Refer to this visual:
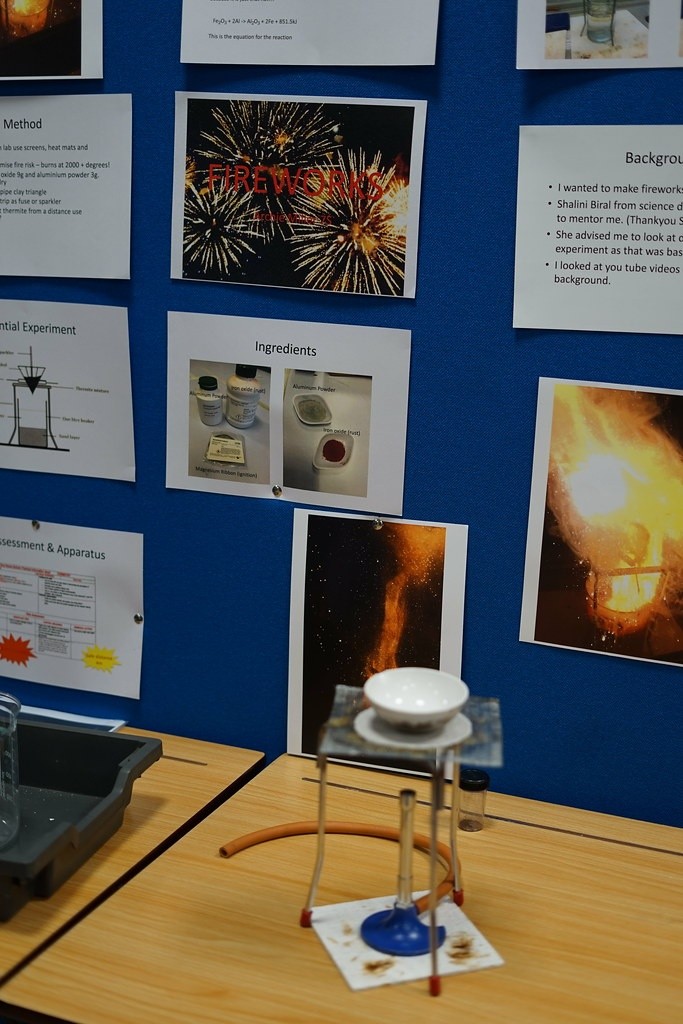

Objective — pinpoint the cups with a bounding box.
[586,0,616,42]
[0,693,24,846]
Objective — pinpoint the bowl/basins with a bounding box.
[365,665,471,735]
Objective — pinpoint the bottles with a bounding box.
[453,767,489,831]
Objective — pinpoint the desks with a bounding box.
[0,698,683,1020]
